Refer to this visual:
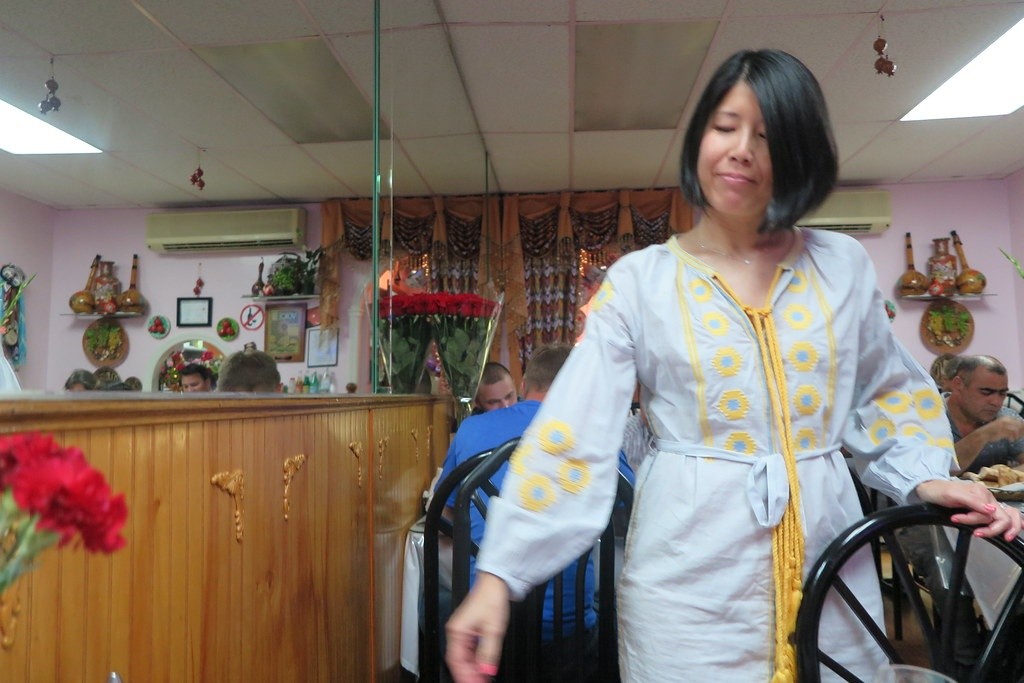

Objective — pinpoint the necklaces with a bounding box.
[695,231,773,264]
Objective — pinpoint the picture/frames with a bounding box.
[175,295,214,329]
[304,324,339,369]
[263,301,308,364]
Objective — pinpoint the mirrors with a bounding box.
[0,0,503,405]
[149,339,229,391]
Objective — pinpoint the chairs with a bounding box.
[790,493,1024,683]
[418,444,617,683]
[453,436,638,683]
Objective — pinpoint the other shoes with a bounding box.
[952,601,986,669]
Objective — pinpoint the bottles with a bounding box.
[91,261,120,314]
[288,367,336,393]
[263,275,276,296]
[927,238,957,296]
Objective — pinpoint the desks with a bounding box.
[403,511,627,683]
[943,481,1024,631]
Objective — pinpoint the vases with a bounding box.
[116,252,148,312]
[927,234,958,294]
[897,230,930,296]
[95,260,119,313]
[67,255,101,313]
[951,224,988,295]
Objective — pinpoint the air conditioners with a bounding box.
[796,189,892,236]
[141,204,307,257]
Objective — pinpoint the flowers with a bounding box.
[366,293,435,394]
[424,290,503,429]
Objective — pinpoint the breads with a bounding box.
[961,463,1024,489]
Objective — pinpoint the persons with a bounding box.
[217,350,282,393]
[64,369,99,392]
[434,342,654,683]
[181,363,213,393]
[98,383,133,391]
[444,49,1024,683]
[894,353,1024,683]
